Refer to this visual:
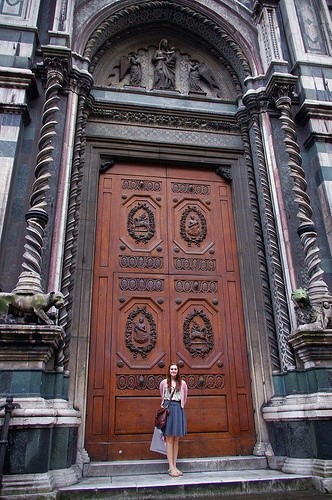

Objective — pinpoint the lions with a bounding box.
[0,291,65,325]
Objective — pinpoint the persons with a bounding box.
[128,38,205,92]
[160,362,188,477]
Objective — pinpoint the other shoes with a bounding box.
[168,467,179,476]
[173,466,183,476]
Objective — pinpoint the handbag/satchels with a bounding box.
[151,426,167,455]
[155,408,170,429]
